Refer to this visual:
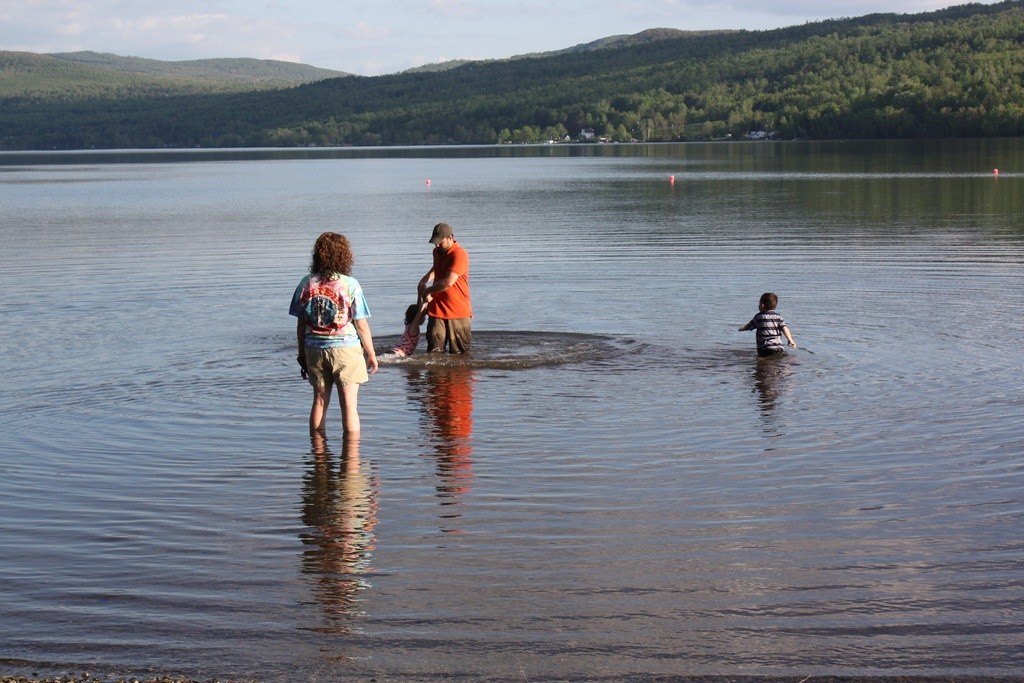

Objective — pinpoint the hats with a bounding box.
[428,222,453,244]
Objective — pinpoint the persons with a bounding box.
[375,283,432,361]
[287,229,381,428]
[736,292,798,358]
[417,223,473,353]
